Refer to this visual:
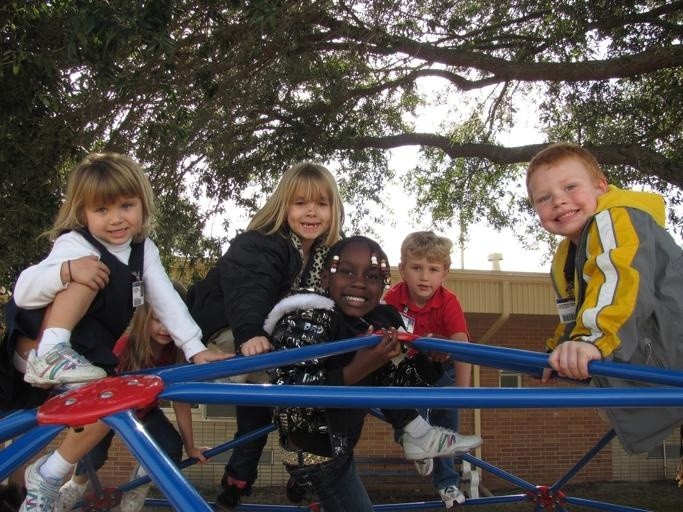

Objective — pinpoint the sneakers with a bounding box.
[120,465,153,512]
[398,426,483,461]
[19,453,61,512]
[56,480,88,512]
[413,459,433,476]
[23,343,106,384]
[215,487,241,512]
[438,485,465,510]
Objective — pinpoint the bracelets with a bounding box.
[65,259,76,284]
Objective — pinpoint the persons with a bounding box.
[380,231,471,510]
[526,144,683,457]
[13,152,236,512]
[188,162,346,512]
[54,279,215,512]
[268,236,482,512]
